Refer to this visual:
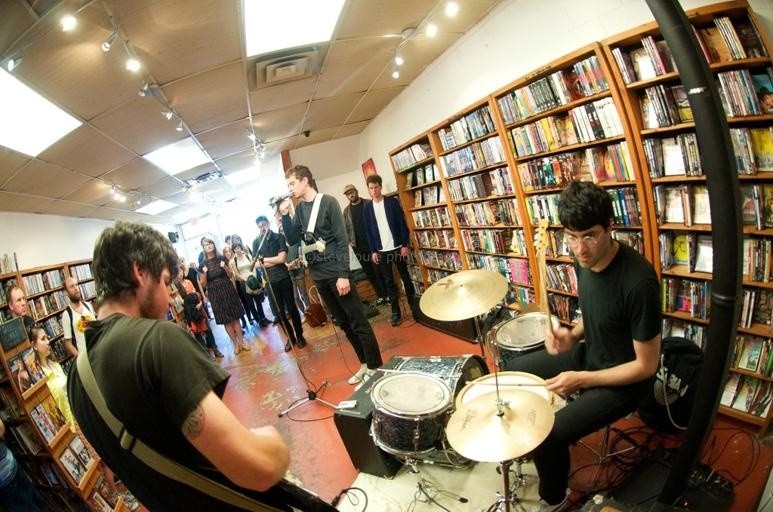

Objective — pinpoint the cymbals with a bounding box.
[419,268,507,321]
[447,389,556,463]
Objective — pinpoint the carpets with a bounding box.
[330,443,546,511]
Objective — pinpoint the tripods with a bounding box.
[260,265,337,417]
[403,466,537,512]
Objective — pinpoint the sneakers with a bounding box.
[375,296,392,307]
[391,313,401,325]
[234,344,251,355]
[207,347,224,361]
[241,317,273,328]
[349,364,378,392]
[530,488,568,512]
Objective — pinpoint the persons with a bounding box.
[27,324,139,511]
[66,221,292,512]
[505,182,664,512]
[172,164,415,392]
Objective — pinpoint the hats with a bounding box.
[343,184,356,194]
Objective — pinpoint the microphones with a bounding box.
[270,192,293,206]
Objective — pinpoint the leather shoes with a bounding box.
[298,337,306,348]
[285,338,296,352]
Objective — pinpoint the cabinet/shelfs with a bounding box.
[389,0,772,437]
[0,254,188,511]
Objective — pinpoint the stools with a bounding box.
[564,386,635,491]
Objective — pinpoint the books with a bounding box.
[0,263,118,512]
[392,0,772,419]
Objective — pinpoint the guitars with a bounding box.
[295,238,326,269]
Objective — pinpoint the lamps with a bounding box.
[386,0,462,84]
[57,2,220,171]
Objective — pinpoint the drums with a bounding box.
[392,355,489,467]
[368,373,451,455]
[494,313,563,359]
[457,371,554,414]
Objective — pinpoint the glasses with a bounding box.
[566,231,603,248]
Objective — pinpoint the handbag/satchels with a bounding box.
[360,299,379,317]
[305,303,327,326]
[637,337,702,429]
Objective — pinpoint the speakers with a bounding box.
[333,354,405,479]
